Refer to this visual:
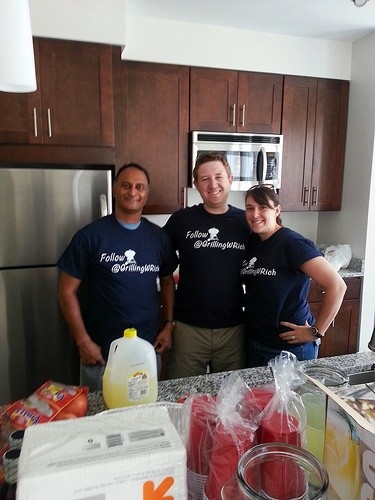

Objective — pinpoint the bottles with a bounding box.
[291,364,349,488]
[103,328,158,409]
[220,442,329,500]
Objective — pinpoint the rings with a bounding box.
[292,336,295,340]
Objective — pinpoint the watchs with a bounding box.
[310,325,324,339]
[164,319,175,328]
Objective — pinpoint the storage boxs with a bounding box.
[14,405,189,500]
[298,370,375,500]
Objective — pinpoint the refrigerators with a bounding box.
[0,168,114,407]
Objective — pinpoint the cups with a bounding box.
[186,387,303,500]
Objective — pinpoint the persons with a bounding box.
[57,162,175,393]
[240,184,346,367]
[161,154,252,380]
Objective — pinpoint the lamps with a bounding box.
[0,0,38,93]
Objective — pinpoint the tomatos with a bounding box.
[51,394,87,420]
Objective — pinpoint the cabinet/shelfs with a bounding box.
[306,277,362,359]
[113,60,351,216]
[0,36,116,171]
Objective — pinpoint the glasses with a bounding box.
[248,183,276,192]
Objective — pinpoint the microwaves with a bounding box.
[188,130,284,192]
